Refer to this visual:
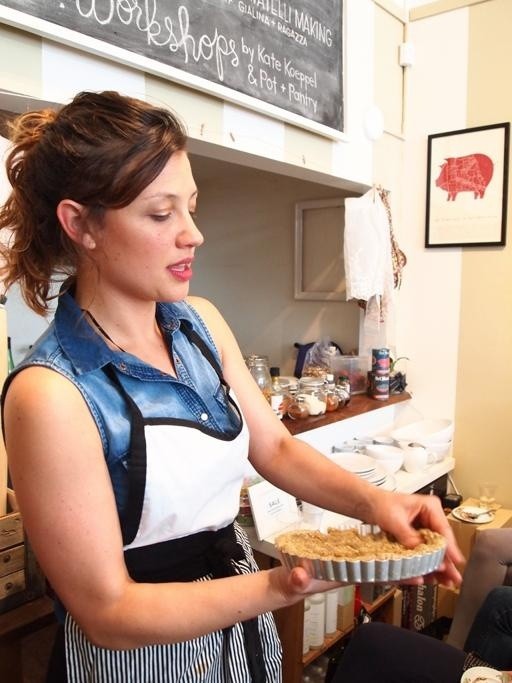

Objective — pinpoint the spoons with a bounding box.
[464,509,499,518]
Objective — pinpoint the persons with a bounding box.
[444,528,512,652]
[324,622,512,683]
[462,587,511,671]
[1,91,467,683]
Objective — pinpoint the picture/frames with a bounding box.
[292,196,354,302]
[423,120,510,248]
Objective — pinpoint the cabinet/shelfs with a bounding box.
[239,452,456,682]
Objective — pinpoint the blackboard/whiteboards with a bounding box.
[1,1,351,140]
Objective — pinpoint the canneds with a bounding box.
[367,348,390,402]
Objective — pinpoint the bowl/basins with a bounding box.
[322,416,453,493]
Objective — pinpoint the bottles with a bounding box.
[1,291,13,397]
[370,347,391,401]
[302,588,337,683]
[248,353,350,420]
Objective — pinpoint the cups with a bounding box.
[332,355,369,395]
[479,495,496,509]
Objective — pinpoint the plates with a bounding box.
[451,505,494,525]
[460,666,502,683]
